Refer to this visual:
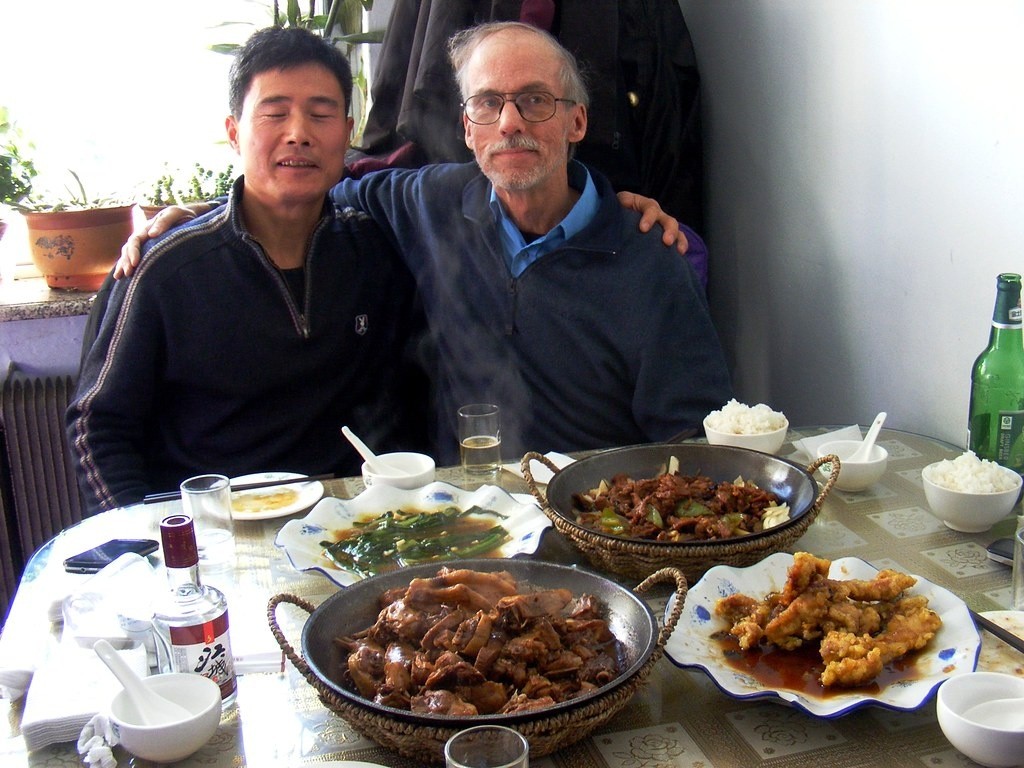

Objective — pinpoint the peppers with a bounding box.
[582,500,748,539]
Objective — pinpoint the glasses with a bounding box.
[460,90,577,125]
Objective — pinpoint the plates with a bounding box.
[273,479,552,590]
[201,471,324,520]
[975,611,1024,682]
[660,552,984,719]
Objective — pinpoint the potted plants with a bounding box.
[142,156,242,222]
[20,179,141,292]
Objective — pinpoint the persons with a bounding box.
[113,19,733,467]
[65,23,689,516]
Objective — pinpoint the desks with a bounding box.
[0,421,1024,768]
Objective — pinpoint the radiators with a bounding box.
[0,376,82,618]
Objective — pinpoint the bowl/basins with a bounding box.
[936,671,1024,768]
[921,462,1023,532]
[361,451,435,489]
[816,440,889,492]
[108,672,222,763]
[703,419,789,456]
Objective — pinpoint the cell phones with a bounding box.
[64,539,160,574]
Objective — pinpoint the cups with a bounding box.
[444,725,529,768]
[457,403,503,474]
[180,475,238,572]
[1010,528,1024,610]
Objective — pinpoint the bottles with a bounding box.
[152,512,238,710]
[965,272,1024,502]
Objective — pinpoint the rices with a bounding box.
[926,449,1019,493]
[706,397,786,434]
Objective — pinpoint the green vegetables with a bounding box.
[319,505,512,577]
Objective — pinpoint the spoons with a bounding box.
[844,412,887,461]
[93,640,192,726]
[341,427,408,477]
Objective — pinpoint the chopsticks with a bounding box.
[968,607,1024,654]
[142,474,334,504]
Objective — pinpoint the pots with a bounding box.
[267,565,688,758]
[518,444,841,585]
